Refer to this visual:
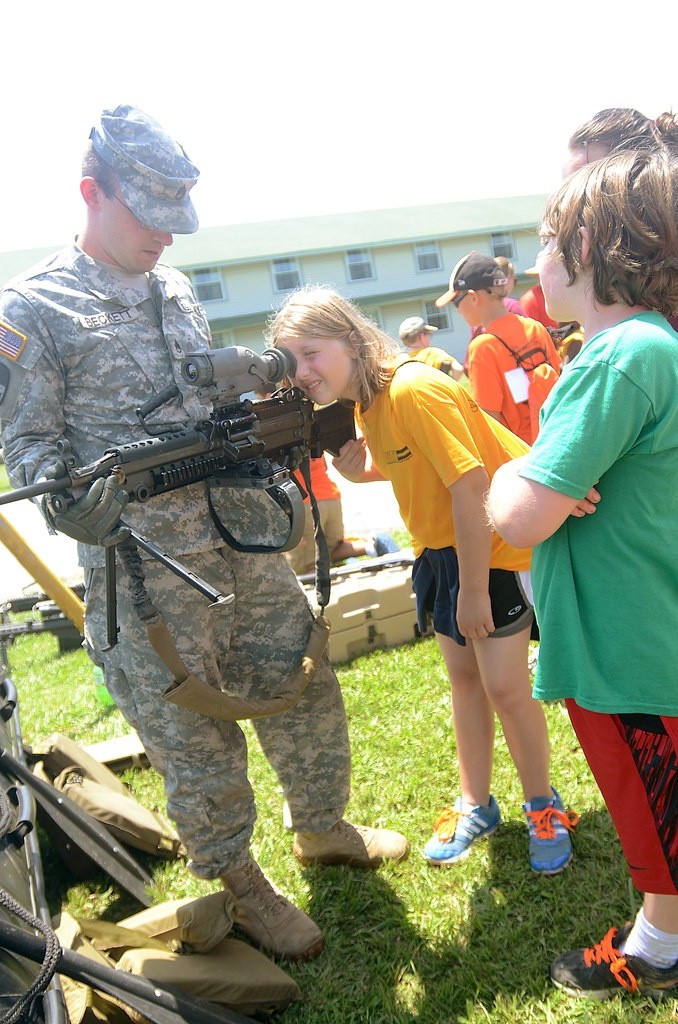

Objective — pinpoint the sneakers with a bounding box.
[425,794,500,864]
[522,787,573,875]
[550,923,678,996]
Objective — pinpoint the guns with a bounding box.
[0,583,84,665]
[0,345,357,652]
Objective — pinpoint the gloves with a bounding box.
[44,466,133,548]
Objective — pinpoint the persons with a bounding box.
[254,378,401,575]
[399,250,583,640]
[483,107,678,1001]
[271,285,579,875]
[0,103,409,964]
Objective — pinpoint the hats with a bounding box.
[434,250,507,309]
[398,317,439,337]
[89,103,200,234]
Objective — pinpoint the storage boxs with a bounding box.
[306,565,436,663]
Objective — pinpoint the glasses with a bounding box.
[94,179,152,232]
[423,332,433,336]
[452,289,479,308]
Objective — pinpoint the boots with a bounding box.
[293,820,412,867]
[221,855,325,959]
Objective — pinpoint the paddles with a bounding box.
[0,745,158,906]
[0,916,254,1024]
